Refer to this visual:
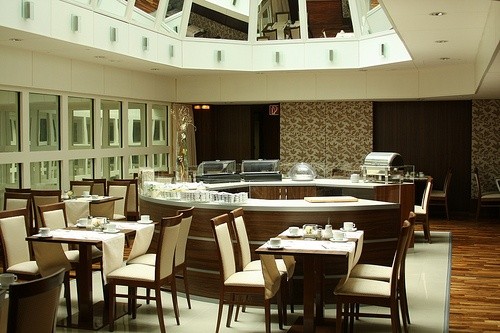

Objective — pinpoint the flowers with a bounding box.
[67,190,74,196]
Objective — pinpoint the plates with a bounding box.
[80,195,91,197]
[329,239,348,242]
[76,223,87,227]
[37,234,52,238]
[137,220,154,224]
[340,228,357,232]
[287,234,301,237]
[267,246,285,249]
[102,230,120,233]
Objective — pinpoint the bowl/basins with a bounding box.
[92,195,98,199]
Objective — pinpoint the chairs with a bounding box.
[474,167,500,216]
[0,178,452,333]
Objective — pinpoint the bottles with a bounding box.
[38,228,50,236]
[288,227,300,236]
[324,225,332,236]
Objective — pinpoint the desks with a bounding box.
[396,177,434,180]
[254,228,365,333]
[25,222,159,331]
[61,195,124,226]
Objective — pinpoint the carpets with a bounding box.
[57,230,451,333]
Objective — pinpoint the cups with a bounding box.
[332,232,345,240]
[140,215,150,222]
[82,191,89,196]
[269,238,282,248]
[343,222,355,231]
[106,224,117,232]
[76,218,88,226]
